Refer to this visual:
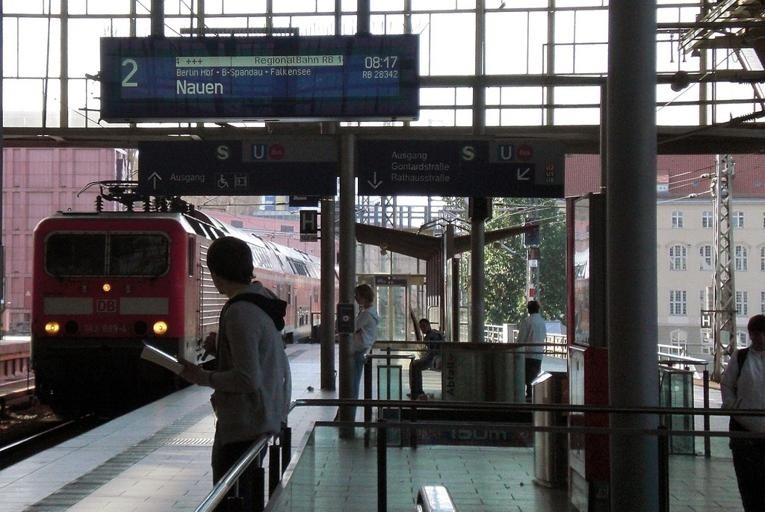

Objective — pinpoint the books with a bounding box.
[137,340,198,385]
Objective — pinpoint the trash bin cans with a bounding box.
[531,371,569,488]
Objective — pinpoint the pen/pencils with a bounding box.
[201,351,209,361]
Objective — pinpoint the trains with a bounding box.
[32,180,339,417]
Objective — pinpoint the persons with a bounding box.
[333,283,379,422]
[513,300,547,403]
[174,234,293,512]
[718,313,765,512]
[405,317,446,401]
